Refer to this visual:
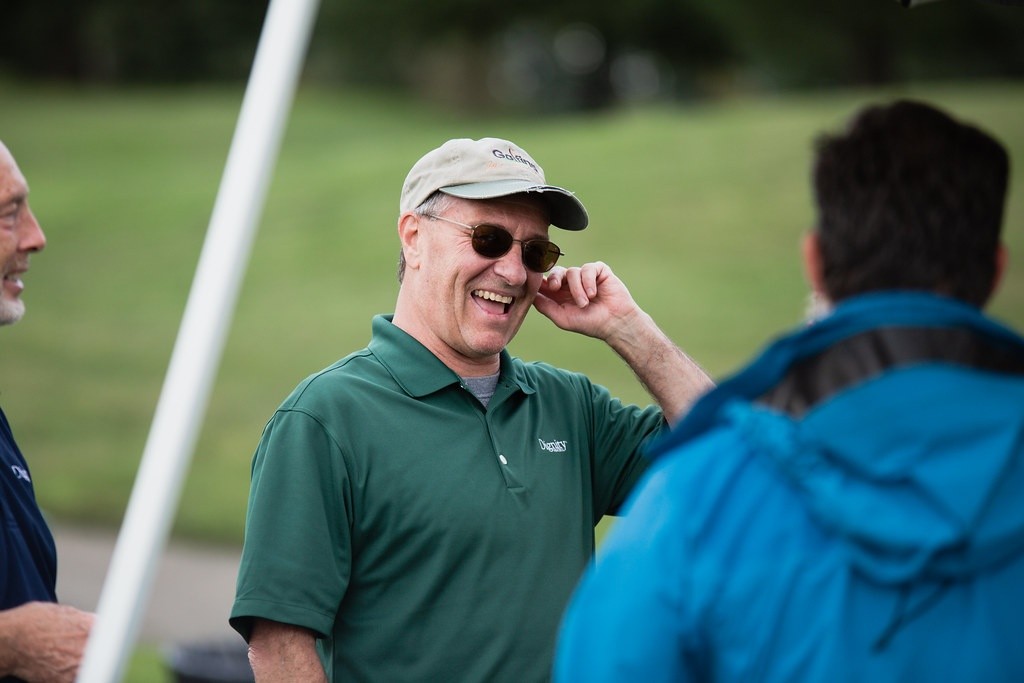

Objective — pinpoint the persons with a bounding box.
[227,138,718,682]
[548,98,1024,682]
[0,141,94,683]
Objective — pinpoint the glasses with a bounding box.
[418,212,566,273]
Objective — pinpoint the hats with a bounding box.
[399,137,588,232]
